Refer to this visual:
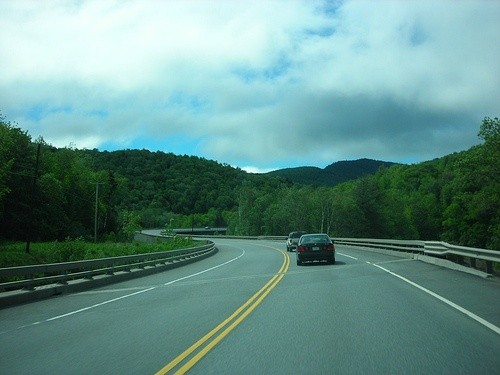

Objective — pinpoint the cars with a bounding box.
[285,231,308,252]
[293,233,335,265]
